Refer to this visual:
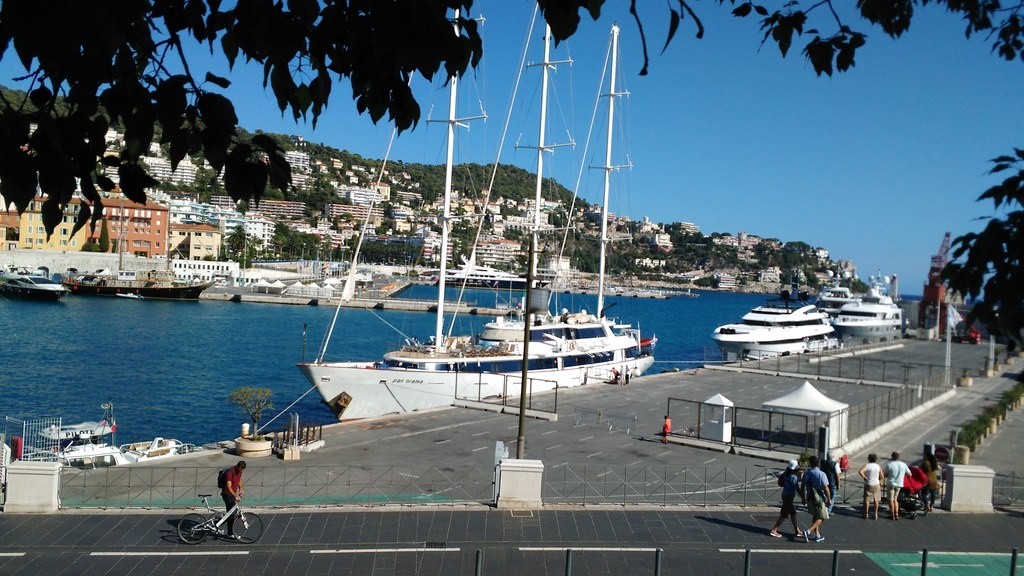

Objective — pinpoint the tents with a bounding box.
[762,381,849,449]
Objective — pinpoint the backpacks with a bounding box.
[218,465,234,488]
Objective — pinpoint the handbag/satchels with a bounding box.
[777,474,785,487]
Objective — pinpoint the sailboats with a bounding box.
[61,199,221,300]
[295,6,655,421]
[474,20,638,346]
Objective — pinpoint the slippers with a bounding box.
[796,532,805,536]
[770,532,782,538]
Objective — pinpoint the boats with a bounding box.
[710,268,841,364]
[0,261,72,302]
[817,272,910,347]
[56,442,131,470]
[38,396,116,439]
[118,437,201,464]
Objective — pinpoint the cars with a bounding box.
[939,324,983,344]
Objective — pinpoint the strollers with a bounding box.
[896,466,930,520]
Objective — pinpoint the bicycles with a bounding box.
[177,494,264,545]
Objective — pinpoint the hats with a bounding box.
[787,459,800,470]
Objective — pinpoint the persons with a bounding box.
[218,461,246,538]
[820,450,840,515]
[411,337,417,345]
[884,451,912,522]
[770,459,806,537]
[919,453,942,512]
[662,415,672,443]
[801,456,833,543]
[612,365,641,384]
[859,453,884,520]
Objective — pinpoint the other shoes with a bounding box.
[897,515,901,520]
[816,536,825,542]
[803,529,810,542]
[228,535,241,539]
[888,515,896,520]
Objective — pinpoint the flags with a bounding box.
[947,304,964,331]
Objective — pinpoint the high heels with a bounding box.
[924,505,933,511]
